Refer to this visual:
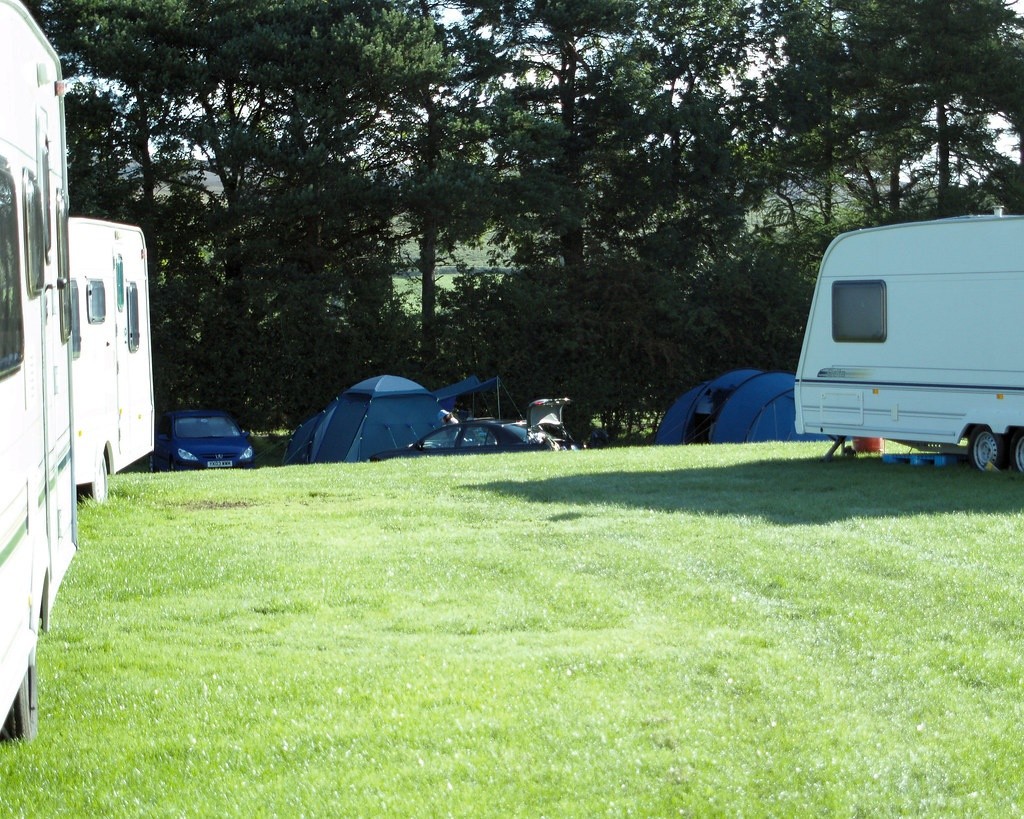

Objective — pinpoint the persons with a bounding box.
[437,410,459,426]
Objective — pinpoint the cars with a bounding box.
[364,418,588,466]
[148,409,255,473]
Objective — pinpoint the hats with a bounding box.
[438,409,449,420]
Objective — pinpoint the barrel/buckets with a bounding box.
[852,436,881,453]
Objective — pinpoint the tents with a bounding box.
[282,375,453,464]
[652,368,853,446]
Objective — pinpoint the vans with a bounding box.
[0,0,83,743]
[793,204,1024,475]
[62,217,156,510]
